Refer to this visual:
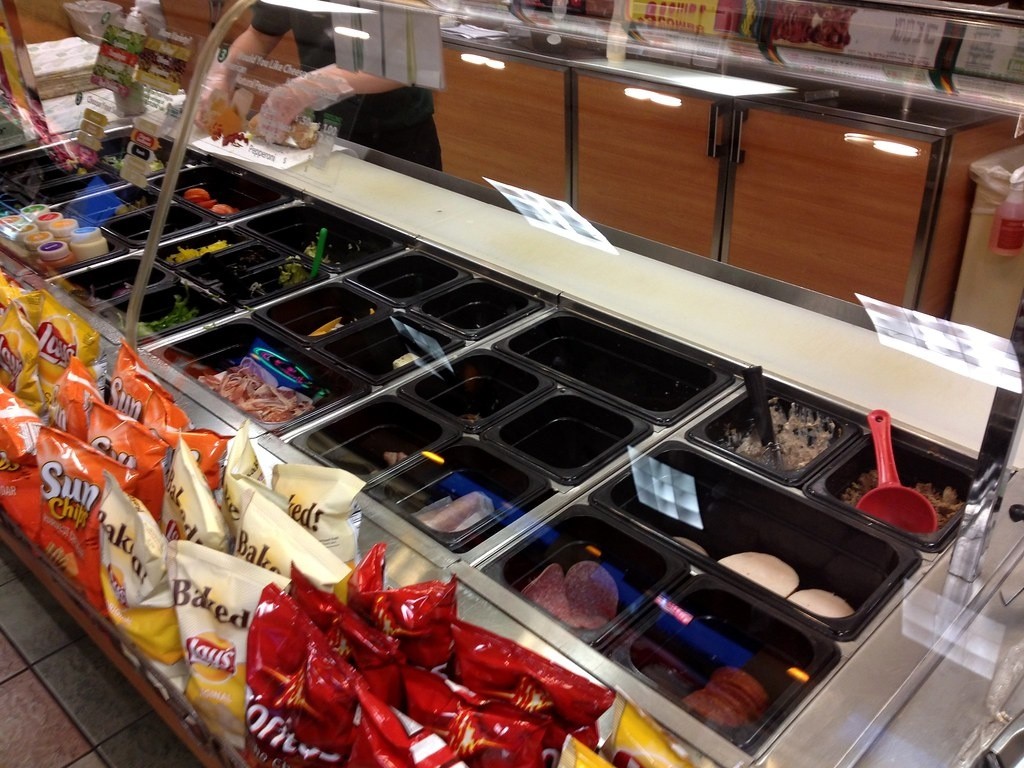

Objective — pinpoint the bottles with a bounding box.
[0,204,112,272]
[989,164,1024,261]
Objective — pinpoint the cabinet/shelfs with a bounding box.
[160,1,325,131]
[428,20,606,216]
[574,45,1024,316]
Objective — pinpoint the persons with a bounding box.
[189,0,444,178]
